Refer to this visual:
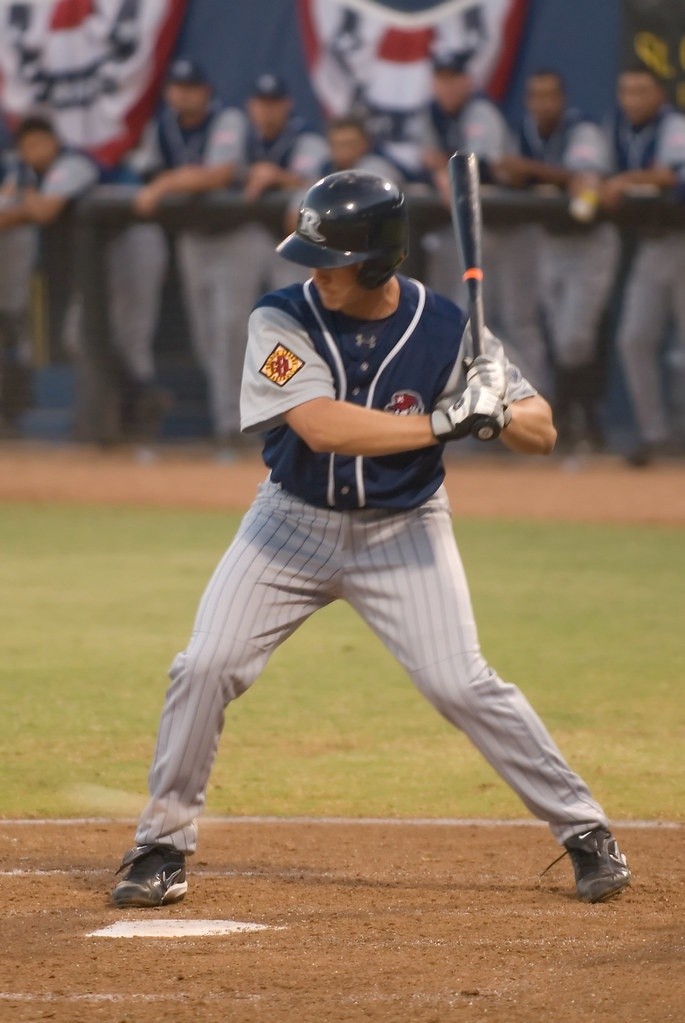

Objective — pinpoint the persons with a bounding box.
[110,170,631,908]
[0,36,685,462]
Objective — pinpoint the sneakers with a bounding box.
[113,843,188,906]
[539,825,632,903]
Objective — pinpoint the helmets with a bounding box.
[275,168,411,289]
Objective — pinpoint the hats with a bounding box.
[254,69,287,100]
[162,60,206,83]
[434,52,465,73]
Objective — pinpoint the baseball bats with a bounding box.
[447,149,501,442]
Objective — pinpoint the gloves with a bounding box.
[429,383,504,443]
[462,354,511,428]
